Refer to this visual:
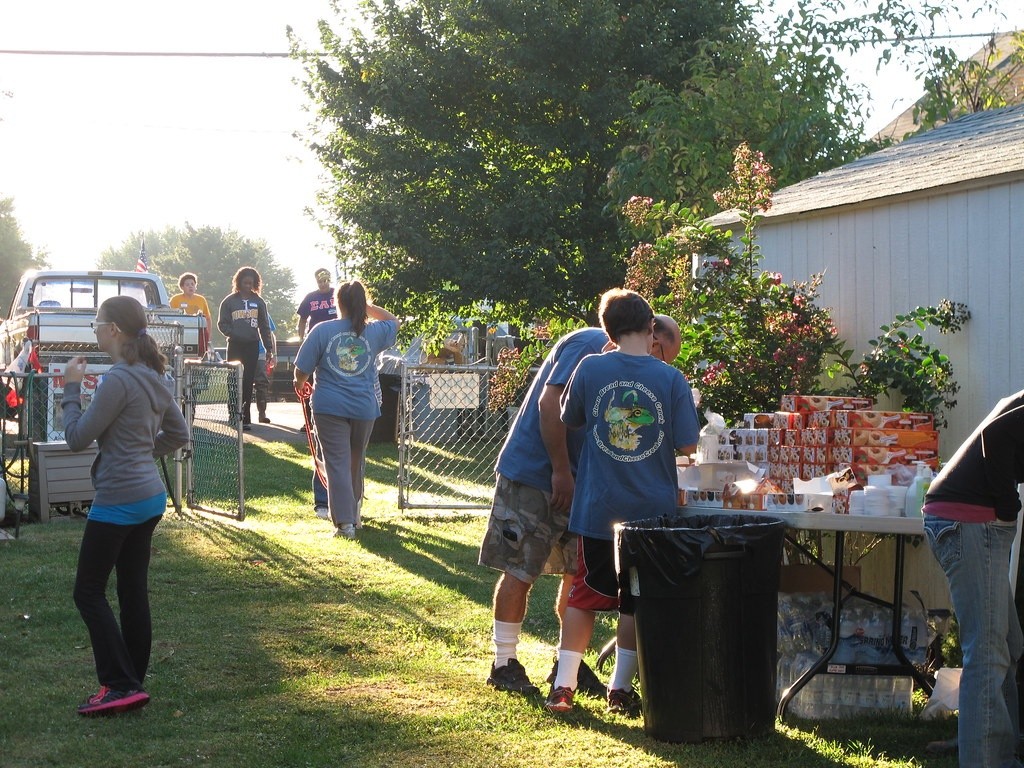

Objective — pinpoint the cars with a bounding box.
[191,339,309,403]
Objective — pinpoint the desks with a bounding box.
[683,507,933,722]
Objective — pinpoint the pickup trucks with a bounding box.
[1,269,210,426]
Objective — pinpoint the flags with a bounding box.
[135,239,149,273]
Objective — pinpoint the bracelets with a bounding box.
[273,353,278,356]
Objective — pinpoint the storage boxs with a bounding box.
[681,394,938,511]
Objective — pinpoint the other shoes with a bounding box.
[300,424,313,432]
[333,525,357,540]
[259,417,270,423]
[243,424,252,430]
[316,506,329,519]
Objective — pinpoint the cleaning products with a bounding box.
[904,459,936,518]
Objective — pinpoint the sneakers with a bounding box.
[545,683,578,713]
[75,686,151,718]
[486,658,540,696]
[607,686,643,715]
[545,656,612,698]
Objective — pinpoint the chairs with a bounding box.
[39,300,61,306]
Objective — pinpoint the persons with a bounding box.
[170,269,338,432]
[479,313,681,706]
[544,287,700,712]
[292,279,400,541]
[920,387,1024,768]
[63,297,190,715]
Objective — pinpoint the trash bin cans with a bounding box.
[613,510,786,744]
[371,373,402,442]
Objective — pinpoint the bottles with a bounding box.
[775,586,930,718]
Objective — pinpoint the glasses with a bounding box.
[90,321,121,334]
